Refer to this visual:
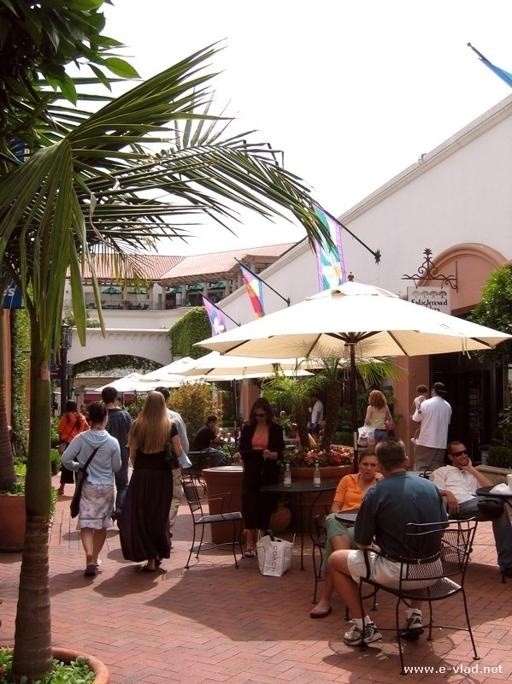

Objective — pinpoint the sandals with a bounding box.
[244,548,255,557]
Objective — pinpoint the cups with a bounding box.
[506,473,512,489]
[227,432,231,442]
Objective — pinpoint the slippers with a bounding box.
[83,561,101,577]
[309,600,333,619]
[144,559,163,572]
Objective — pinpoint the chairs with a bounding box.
[179,440,511,677]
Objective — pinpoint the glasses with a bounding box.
[451,450,468,457]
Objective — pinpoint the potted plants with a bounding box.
[0,1,337,684]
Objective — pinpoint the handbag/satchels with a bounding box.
[257,529,297,578]
[384,416,396,431]
[70,483,81,517]
[476,492,506,514]
[163,441,180,468]
[58,442,67,452]
[357,426,376,445]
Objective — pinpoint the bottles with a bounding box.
[283,463,292,487]
[312,461,321,487]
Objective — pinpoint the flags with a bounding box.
[241,268,265,317]
[202,298,226,338]
[301,204,346,292]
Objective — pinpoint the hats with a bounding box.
[433,382,447,393]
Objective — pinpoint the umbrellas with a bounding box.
[143,355,219,416]
[103,371,168,397]
[166,344,395,424]
[188,279,511,428]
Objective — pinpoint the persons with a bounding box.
[409,384,430,438]
[192,414,225,466]
[118,390,183,571]
[79,401,88,416]
[309,389,325,431]
[411,381,452,470]
[97,385,131,526]
[310,447,384,616]
[151,386,193,539]
[59,399,88,499]
[279,410,288,418]
[364,390,393,440]
[232,396,285,556]
[431,439,511,578]
[64,407,123,576]
[327,436,449,645]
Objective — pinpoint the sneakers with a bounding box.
[342,621,384,647]
[400,609,426,637]
